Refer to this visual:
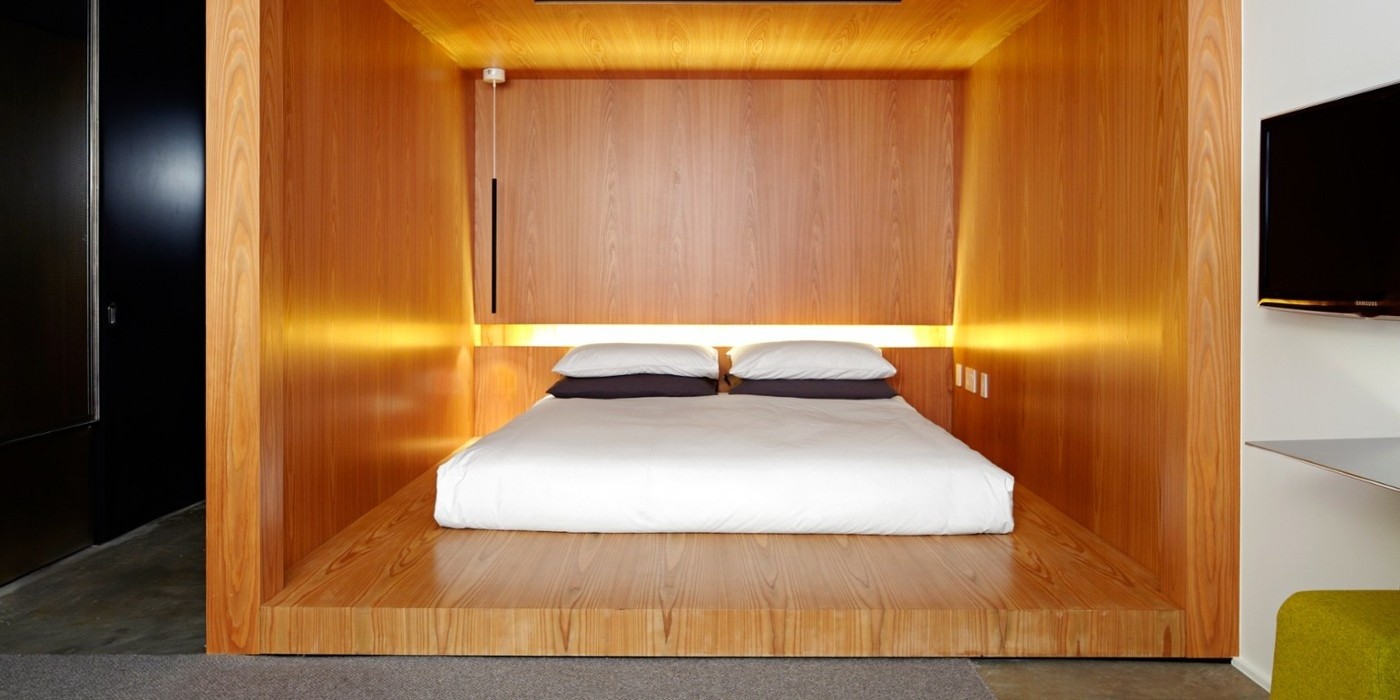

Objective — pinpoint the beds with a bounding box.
[433,344,1015,535]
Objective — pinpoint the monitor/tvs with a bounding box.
[1260,79,1400,319]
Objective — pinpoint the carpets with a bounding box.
[0,653,998,700]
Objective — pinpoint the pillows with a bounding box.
[545,376,718,398]
[725,339,897,380]
[728,378,899,399]
[552,344,720,379]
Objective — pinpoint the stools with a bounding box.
[1269,590,1400,700]
